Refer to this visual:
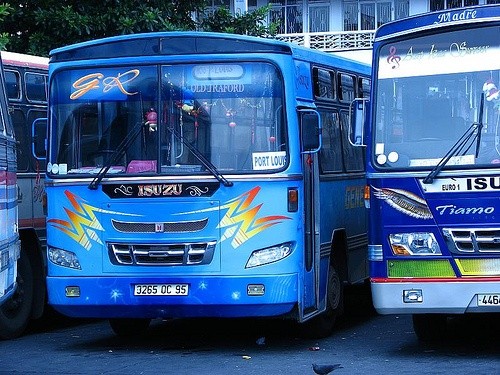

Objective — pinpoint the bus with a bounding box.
[31,30,371,339]
[348,2,500,339]
[0,50,51,339]
